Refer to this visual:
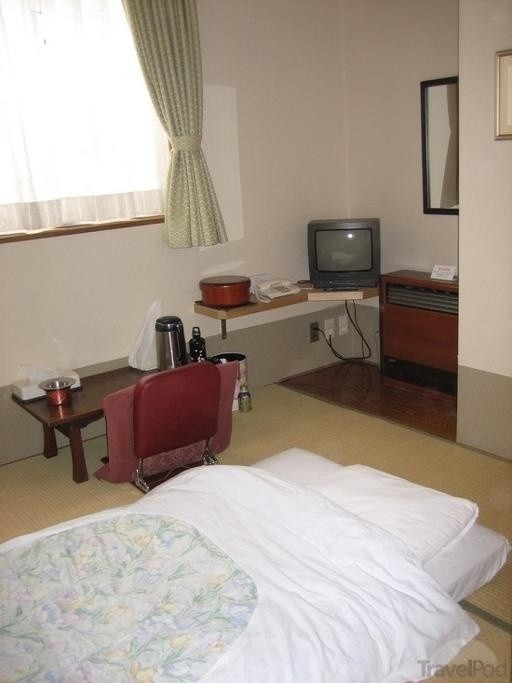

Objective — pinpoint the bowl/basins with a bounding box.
[37,376,78,407]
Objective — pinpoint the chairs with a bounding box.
[134,360,222,493]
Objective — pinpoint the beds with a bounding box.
[0,446,510,682]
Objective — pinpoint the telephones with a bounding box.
[254,277,300,303]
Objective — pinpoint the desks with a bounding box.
[11,352,220,484]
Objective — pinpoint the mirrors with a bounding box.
[421,76,459,214]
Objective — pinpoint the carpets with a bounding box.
[275,358,457,443]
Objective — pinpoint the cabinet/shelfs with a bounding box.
[380,269,458,398]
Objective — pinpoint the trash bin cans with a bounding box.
[210,353,247,412]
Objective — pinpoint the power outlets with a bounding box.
[310,318,336,342]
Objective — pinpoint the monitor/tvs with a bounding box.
[307,217,380,291]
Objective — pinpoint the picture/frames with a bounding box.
[495,49,512,140]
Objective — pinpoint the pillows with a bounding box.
[310,464,480,565]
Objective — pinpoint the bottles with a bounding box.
[237,384,252,412]
[154,314,188,372]
[188,326,207,365]
